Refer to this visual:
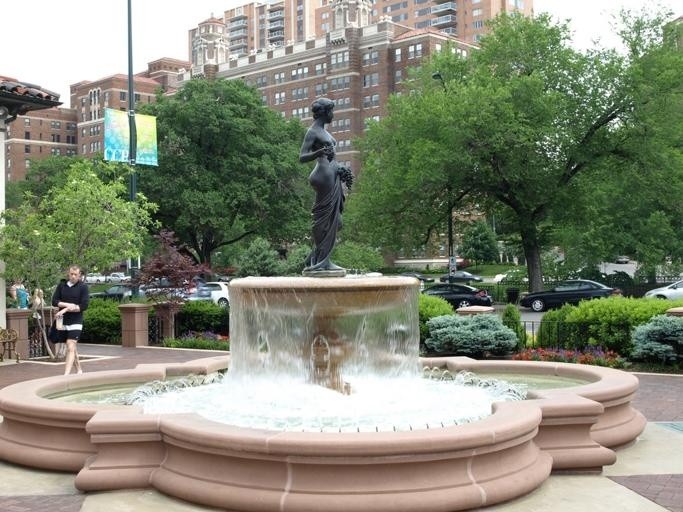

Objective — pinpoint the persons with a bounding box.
[298,95,354,272]
[29,288,46,348]
[11,275,28,309]
[50,264,89,375]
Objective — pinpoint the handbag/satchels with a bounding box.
[49,314,67,344]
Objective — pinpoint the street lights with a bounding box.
[431,72,456,283]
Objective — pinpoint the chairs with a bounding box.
[0,327,21,363]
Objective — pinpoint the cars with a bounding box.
[81,272,229,308]
[397,270,495,310]
[519,256,683,312]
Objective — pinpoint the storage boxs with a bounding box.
[505,287,520,305]
